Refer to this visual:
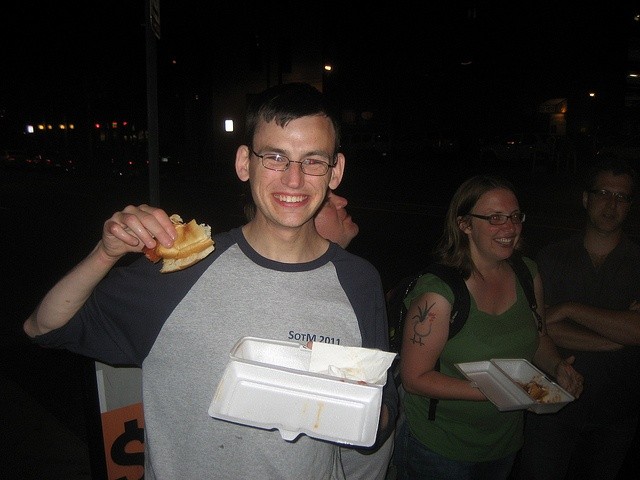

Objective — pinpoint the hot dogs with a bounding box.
[141,213,215,273]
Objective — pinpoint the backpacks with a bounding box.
[386,245,545,422]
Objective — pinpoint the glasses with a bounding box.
[588,189,630,204]
[464,211,525,226]
[250,147,338,177]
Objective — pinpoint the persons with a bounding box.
[392,173,585,480]
[23,83,399,479]
[521,156,639,479]
[229,170,408,479]
